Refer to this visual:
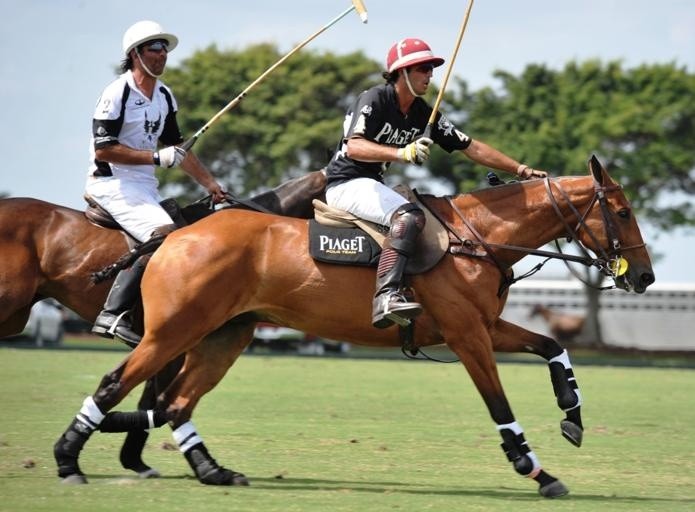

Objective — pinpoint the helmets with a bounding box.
[387,38,444,73]
[122,21,178,56]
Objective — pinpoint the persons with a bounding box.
[321,36,553,330]
[82,17,229,348]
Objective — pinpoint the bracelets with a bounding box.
[517,163,528,177]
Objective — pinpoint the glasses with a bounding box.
[142,41,168,52]
[400,64,433,72]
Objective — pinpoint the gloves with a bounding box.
[398,137,433,163]
[154,145,186,168]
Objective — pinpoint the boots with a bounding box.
[92,224,183,348]
[372,202,424,328]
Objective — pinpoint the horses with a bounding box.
[0,167,327,479]
[53,154,655,498]
